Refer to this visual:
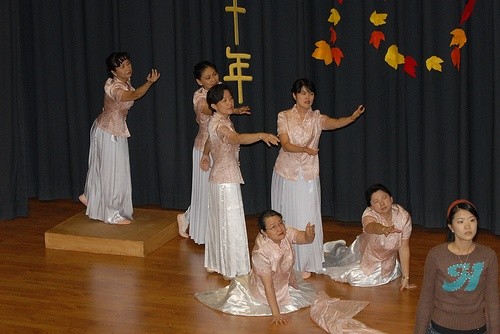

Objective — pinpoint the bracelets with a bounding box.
[402,276,409,280]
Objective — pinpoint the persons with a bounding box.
[192,210,318,326]
[197,85,281,281]
[269,78,365,277]
[413,200,500,334]
[79,53,160,224]
[321,184,417,292]
[177,60,251,245]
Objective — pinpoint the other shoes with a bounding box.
[323,239,346,253]
[79,194,88,207]
[116,218,132,225]
[302,272,312,279]
[177,214,189,238]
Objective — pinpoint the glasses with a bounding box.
[265,220,285,231]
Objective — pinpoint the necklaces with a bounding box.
[455,244,470,270]
